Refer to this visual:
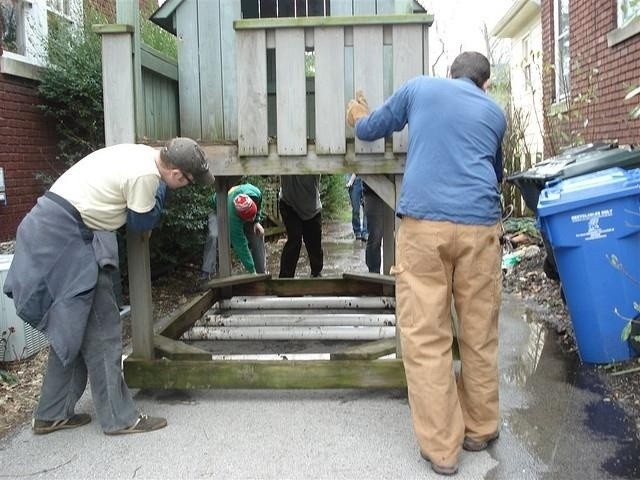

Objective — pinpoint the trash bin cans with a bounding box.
[506,141,640,365]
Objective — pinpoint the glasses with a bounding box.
[182,172,196,185]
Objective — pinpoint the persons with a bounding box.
[346,175,368,244]
[1,136,218,440]
[200,183,270,282]
[275,176,329,281]
[360,173,384,275]
[345,50,508,480]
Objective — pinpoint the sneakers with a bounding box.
[104,414,167,433]
[462,427,500,450]
[421,450,459,474]
[356,232,368,240]
[33,413,90,432]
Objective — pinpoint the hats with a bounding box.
[234,194,257,219]
[166,138,215,185]
